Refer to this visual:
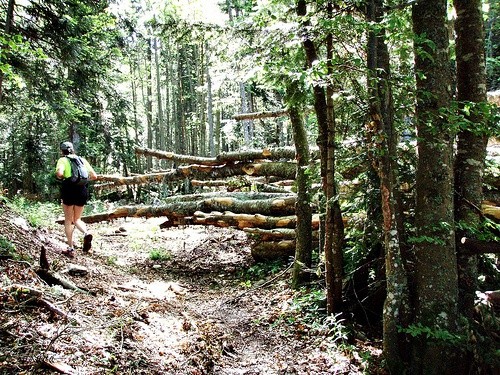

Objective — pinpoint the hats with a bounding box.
[61,142,73,151]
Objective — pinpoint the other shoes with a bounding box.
[82,232,93,252]
[62,247,75,256]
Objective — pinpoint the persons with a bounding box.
[55,141,97,258]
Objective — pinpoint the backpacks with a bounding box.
[63,154,90,186]
